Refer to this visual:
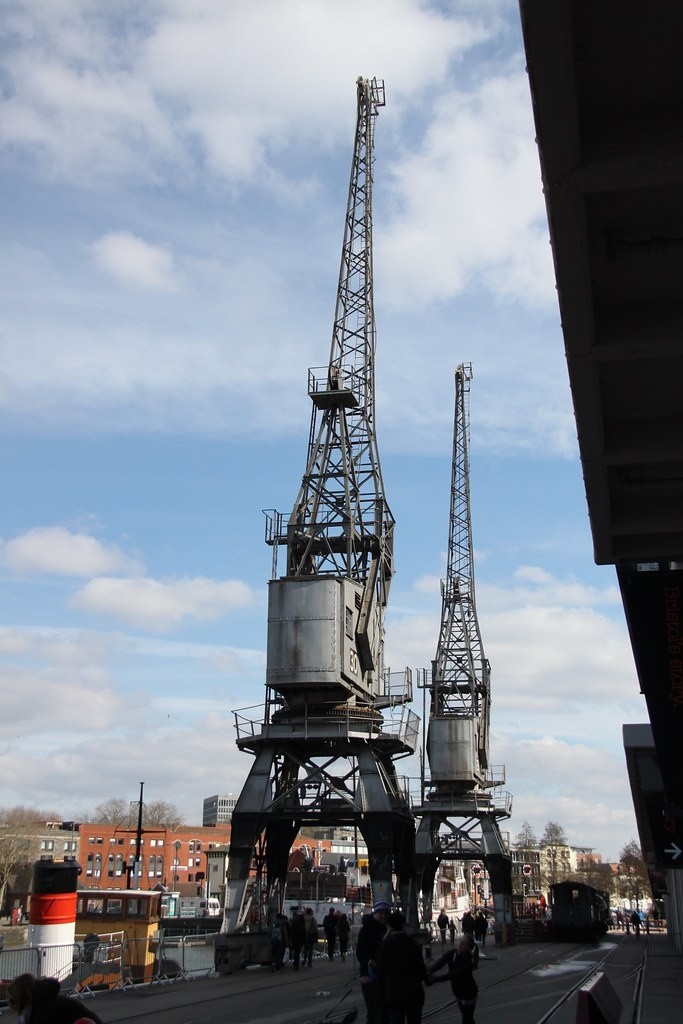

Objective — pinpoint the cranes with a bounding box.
[207,74,418,974]
[403,360,521,949]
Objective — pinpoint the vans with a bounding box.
[195,898,221,917]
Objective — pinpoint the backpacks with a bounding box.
[270,926,282,942]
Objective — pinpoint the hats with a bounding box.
[387,910,407,931]
[374,898,392,913]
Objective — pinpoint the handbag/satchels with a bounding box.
[366,965,382,999]
[308,933,318,943]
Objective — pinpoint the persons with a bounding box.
[356,899,430,1024]
[449,920,458,944]
[539,893,546,924]
[457,912,488,947]
[437,909,450,944]
[323,908,350,961]
[7,974,104,1024]
[271,906,319,970]
[17,904,24,925]
[426,934,479,1024]
[607,910,651,936]
[339,856,346,872]
